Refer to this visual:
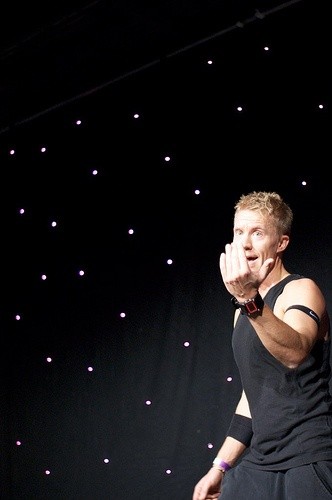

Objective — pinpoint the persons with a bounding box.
[190,190,332,500]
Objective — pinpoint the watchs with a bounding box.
[230,290,264,319]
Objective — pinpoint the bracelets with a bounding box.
[212,458,231,471]
